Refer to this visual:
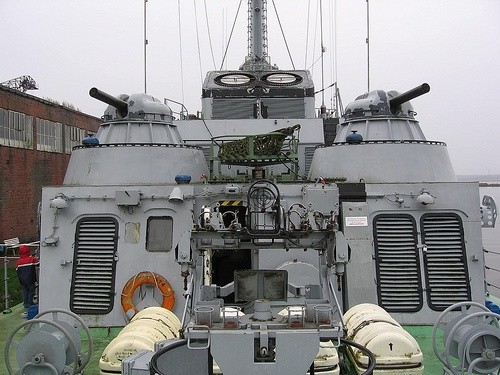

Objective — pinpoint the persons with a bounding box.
[15,245,40,308]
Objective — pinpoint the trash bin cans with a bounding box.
[28,306,38,320]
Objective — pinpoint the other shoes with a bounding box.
[23,302,36,307]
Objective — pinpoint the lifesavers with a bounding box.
[120,270,175,320]
